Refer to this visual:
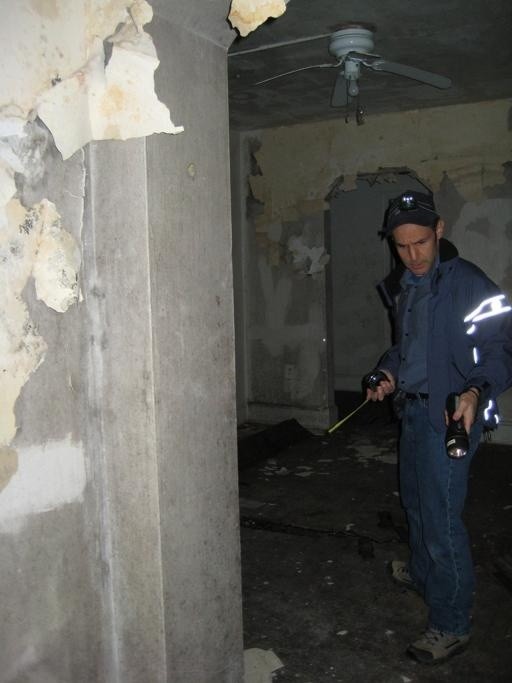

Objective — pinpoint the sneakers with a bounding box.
[406,626,470,665]
[387,559,420,591]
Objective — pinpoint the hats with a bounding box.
[384,189,439,239]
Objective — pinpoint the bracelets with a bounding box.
[470,389,479,399]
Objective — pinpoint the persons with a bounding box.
[359,188,511,668]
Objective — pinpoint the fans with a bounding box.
[252,26,456,108]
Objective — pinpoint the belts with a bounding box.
[400,392,428,401]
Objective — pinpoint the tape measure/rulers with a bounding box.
[327,371,389,434]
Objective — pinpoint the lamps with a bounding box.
[344,57,360,96]
